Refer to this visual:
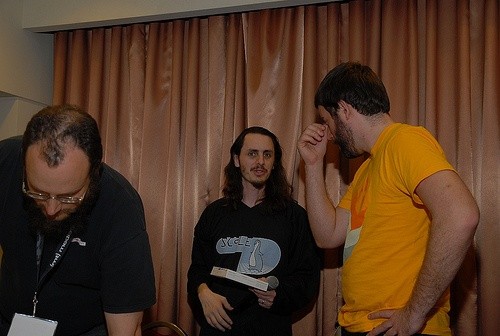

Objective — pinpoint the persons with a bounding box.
[296,61,480,336]
[186,126,323,336]
[0,104,156,336]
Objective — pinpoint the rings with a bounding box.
[261,299,265,305]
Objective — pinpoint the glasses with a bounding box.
[22,166,94,204]
